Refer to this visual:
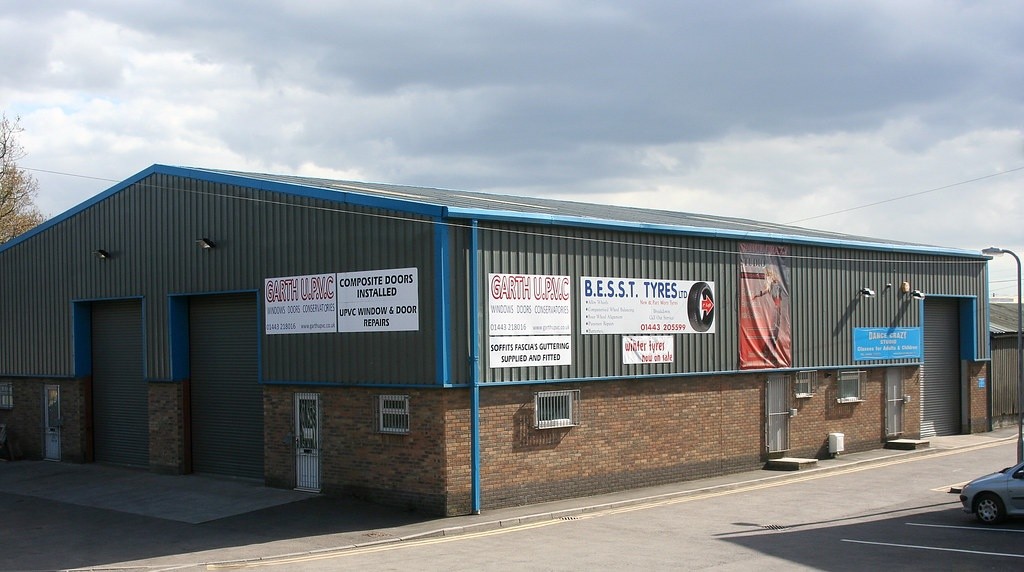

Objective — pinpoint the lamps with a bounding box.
[91,249,110,259]
[860,288,875,298]
[910,290,925,299]
[195,238,216,250]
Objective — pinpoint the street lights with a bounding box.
[983,247,1024,465]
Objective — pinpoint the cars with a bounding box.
[960,461,1024,524]
